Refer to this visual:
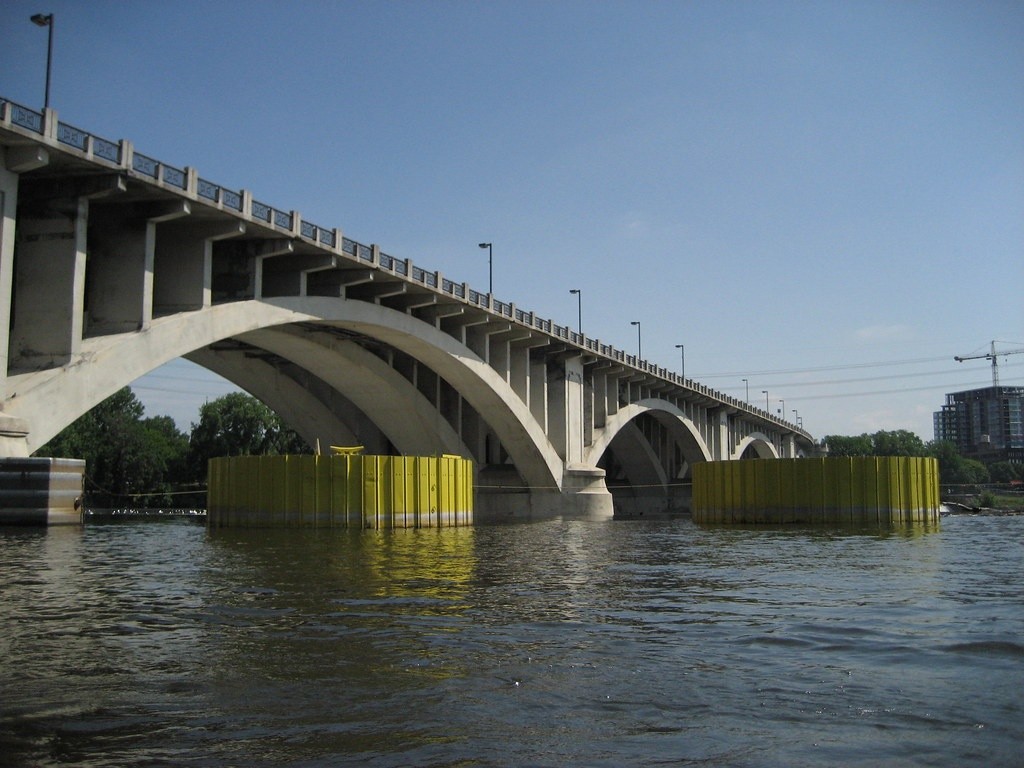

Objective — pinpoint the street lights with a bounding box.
[792,409,802,428]
[29,11,55,108]
[778,400,785,420]
[742,378,749,404]
[569,289,581,334]
[675,344,685,377]
[630,322,642,359]
[478,241,494,293]
[761,390,769,412]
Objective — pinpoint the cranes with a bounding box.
[953,339,1024,386]
